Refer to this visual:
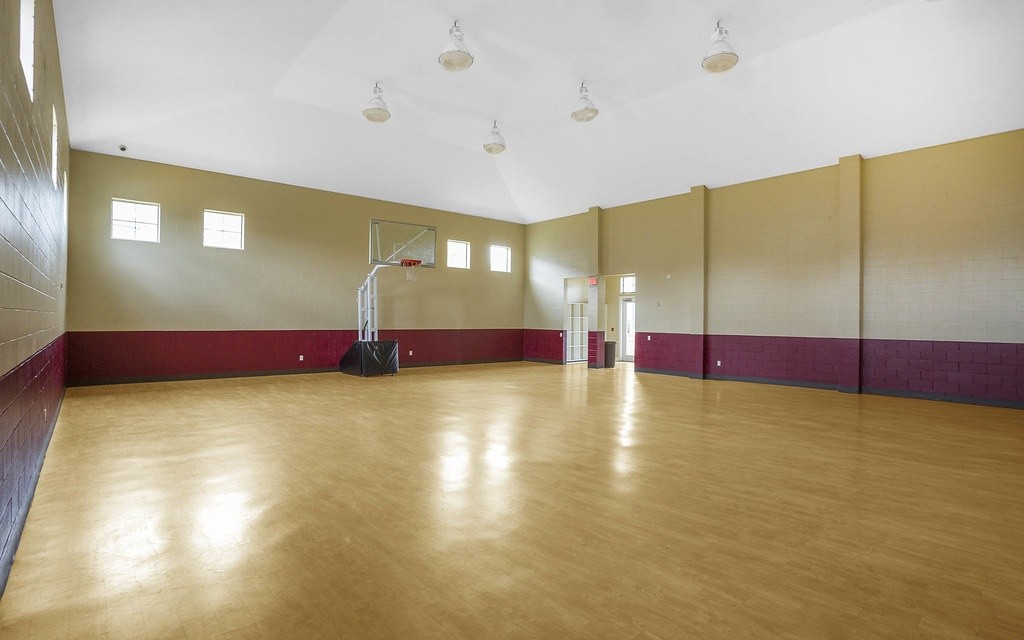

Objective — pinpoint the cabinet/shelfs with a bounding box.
[567,303,589,362]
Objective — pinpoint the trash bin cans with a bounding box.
[604,340,618,368]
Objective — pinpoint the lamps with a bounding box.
[362,82,391,122]
[483,121,506,153]
[438,20,473,71]
[701,21,740,72]
[570,81,599,121]
